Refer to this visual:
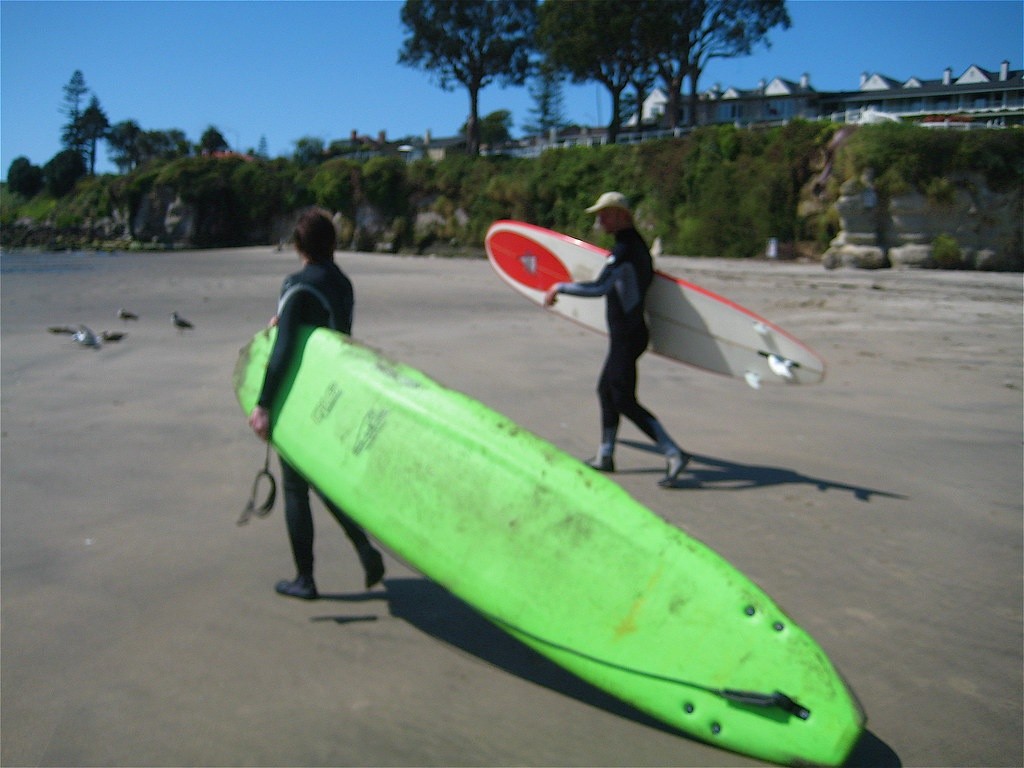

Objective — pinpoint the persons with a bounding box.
[248,208,384,599]
[544,192,690,487]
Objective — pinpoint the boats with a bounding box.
[482,218,828,393]
[232,324,870,768]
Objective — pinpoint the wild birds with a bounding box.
[170,310,195,335]
[117,308,139,327]
[99,330,128,344]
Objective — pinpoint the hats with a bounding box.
[585,191,628,214]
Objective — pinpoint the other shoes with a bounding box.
[584,456,614,471]
[365,549,385,586]
[657,450,693,486]
[277,580,317,600]
